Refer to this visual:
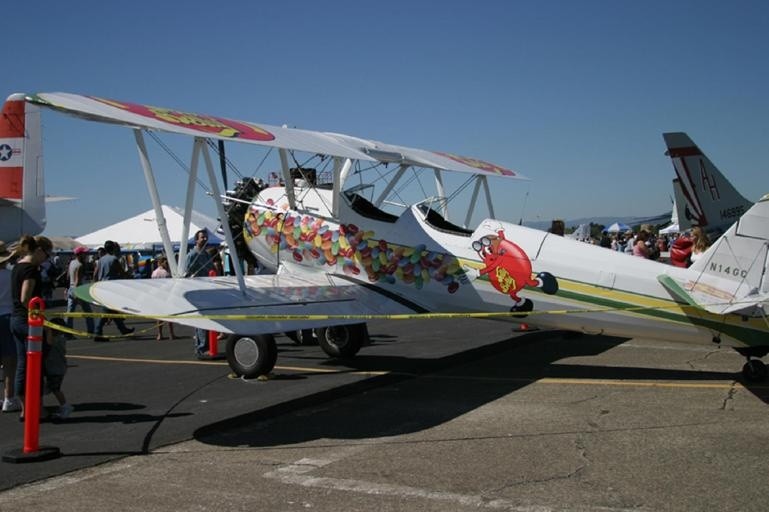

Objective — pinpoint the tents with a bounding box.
[153,226,229,260]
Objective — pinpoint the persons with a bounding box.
[63,240,177,340]
[1,234,73,422]
[184,230,228,362]
[550,219,715,269]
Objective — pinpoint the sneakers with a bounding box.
[19,413,59,423]
[2,399,20,412]
[59,403,74,419]
[94,336,109,342]
[121,327,135,335]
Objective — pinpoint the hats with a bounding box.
[74,245,93,255]
[0,240,18,264]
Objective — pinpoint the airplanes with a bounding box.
[25,90,769,380]
[0,91,47,244]
[661,131,757,249]
